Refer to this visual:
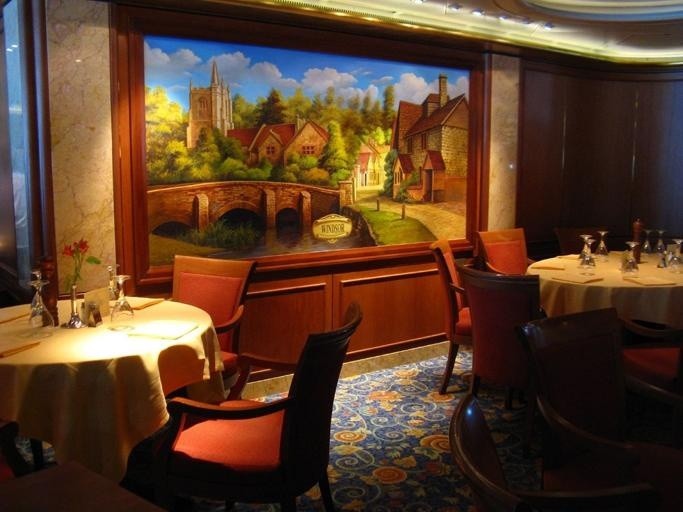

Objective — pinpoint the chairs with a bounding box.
[166,253,258,402]
[430,229,546,393]
[2,418,162,511]
[449,391,663,510]
[171,298,363,511]
[621,344,682,407]
[519,307,682,509]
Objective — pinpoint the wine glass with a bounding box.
[596,230,611,262]
[578,233,596,275]
[659,238,683,274]
[103,264,136,331]
[622,241,640,282]
[641,229,664,253]
[24,268,54,338]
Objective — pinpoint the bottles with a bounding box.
[633,218,641,265]
[78,302,103,327]
[38,257,60,326]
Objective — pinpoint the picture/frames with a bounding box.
[129,3,483,286]
